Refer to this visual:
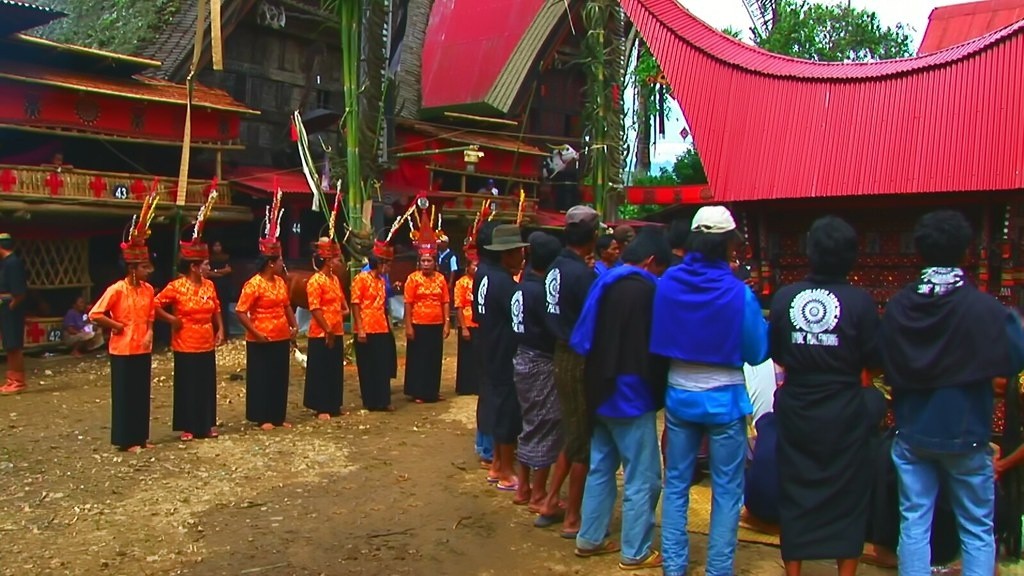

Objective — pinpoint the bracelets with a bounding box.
[293,327,298,331]
[445,318,451,321]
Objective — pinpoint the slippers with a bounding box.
[619,549,663,569]
[487,474,500,481]
[534,508,563,527]
[496,476,532,490]
[513,494,547,504]
[575,540,620,556]
[561,526,610,539]
[480,461,492,468]
[180,431,218,442]
[527,500,564,513]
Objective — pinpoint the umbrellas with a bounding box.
[299,108,343,151]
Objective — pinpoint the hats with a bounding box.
[317,179,341,259]
[259,177,285,256]
[482,223,531,250]
[179,175,219,260]
[435,212,449,242]
[373,190,424,260]
[120,176,161,263]
[528,231,558,269]
[463,199,495,262]
[692,205,746,246]
[565,206,599,230]
[0,233,12,239]
[408,198,437,256]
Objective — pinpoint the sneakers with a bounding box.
[0,379,26,394]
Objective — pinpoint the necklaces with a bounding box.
[125,277,139,307]
[259,272,279,295]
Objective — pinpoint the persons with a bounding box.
[436,234,458,329]
[236,237,300,429]
[402,243,451,404]
[648,206,770,576]
[89,246,155,451]
[475,205,669,571]
[454,246,480,396]
[350,240,402,414]
[872,209,1024,576]
[476,179,499,196]
[739,357,783,524]
[41,154,74,169]
[769,217,889,576]
[0,233,27,395]
[63,295,104,357]
[304,236,349,421]
[208,238,232,295]
[431,172,446,191]
[154,240,225,440]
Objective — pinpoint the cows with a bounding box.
[541,140,580,180]
